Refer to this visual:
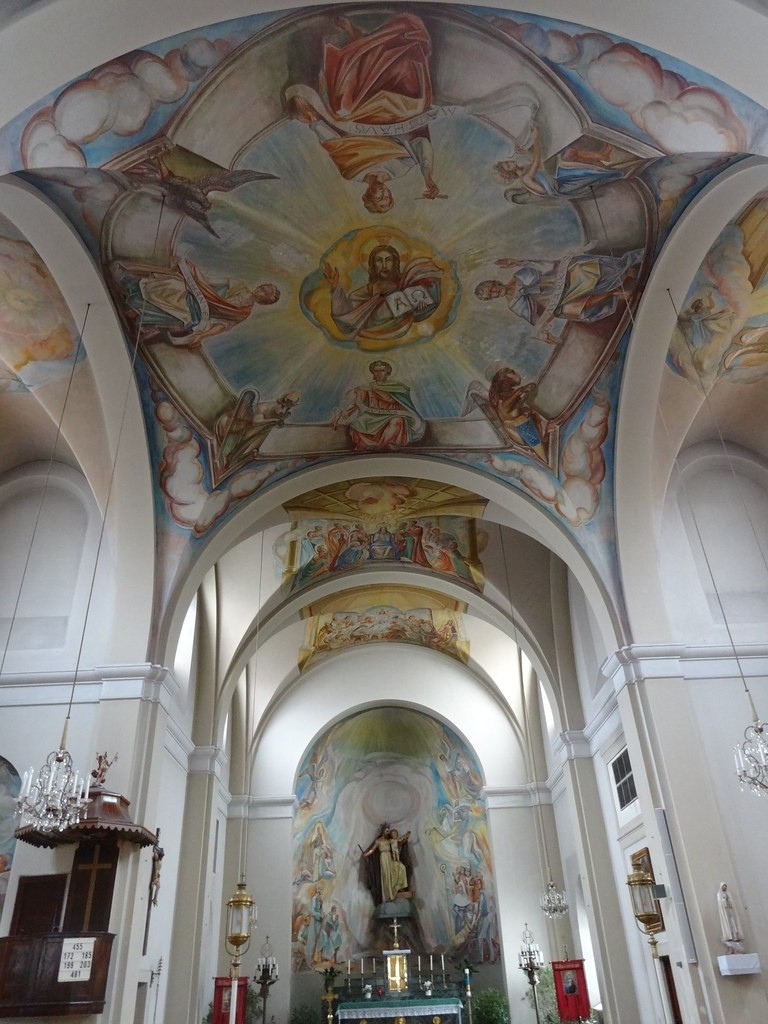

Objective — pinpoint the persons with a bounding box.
[96,752,113,783]
[151,850,165,908]
[716,879,748,954]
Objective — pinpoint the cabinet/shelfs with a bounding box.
[337,976,463,1024]
[0,932,118,1019]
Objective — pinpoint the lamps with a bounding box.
[223,880,256,1024]
[623,859,674,1024]
[589,184,768,796]
[15,194,165,832]
[498,523,571,920]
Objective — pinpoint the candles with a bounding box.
[418,956,421,971]
[464,968,470,991]
[441,955,445,970]
[430,955,433,970]
[347,960,350,974]
[360,958,363,974]
[518,944,544,966]
[253,957,279,977]
[373,958,376,974]
